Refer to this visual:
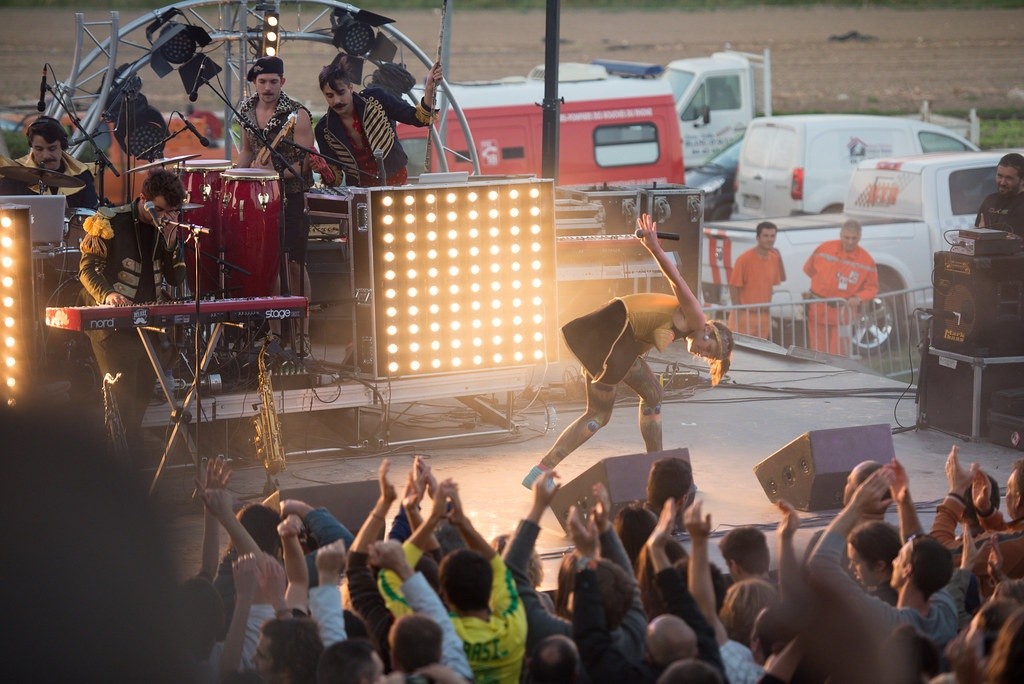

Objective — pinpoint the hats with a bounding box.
[648,458,697,495]
[247,56,284,82]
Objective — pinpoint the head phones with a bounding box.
[27,119,68,149]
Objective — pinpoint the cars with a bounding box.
[684,138,741,220]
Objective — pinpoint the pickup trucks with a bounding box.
[703,148,1024,353]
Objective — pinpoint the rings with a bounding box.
[946,462,950,464]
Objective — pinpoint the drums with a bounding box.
[218,167,282,297]
[53,206,98,274]
[172,158,233,297]
[43,274,186,383]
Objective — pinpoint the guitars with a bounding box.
[251,110,298,168]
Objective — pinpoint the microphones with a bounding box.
[178,112,209,147]
[916,308,955,320]
[144,201,164,234]
[635,228,680,240]
[374,148,387,186]
[67,131,101,146]
[189,57,207,102]
[37,67,47,112]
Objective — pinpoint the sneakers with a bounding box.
[521,465,559,497]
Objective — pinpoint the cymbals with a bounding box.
[123,153,202,175]
[1,165,86,189]
[181,203,204,209]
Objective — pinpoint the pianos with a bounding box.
[42,294,310,501]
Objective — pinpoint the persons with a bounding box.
[74,169,186,464]
[307,60,444,189]
[172,444,1024,684]
[0,115,98,398]
[726,222,786,342]
[974,153,1024,254]
[234,57,315,361]
[523,213,734,494]
[802,219,880,358]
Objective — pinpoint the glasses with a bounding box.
[909,531,936,553]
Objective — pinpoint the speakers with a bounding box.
[261,480,386,540]
[754,424,895,512]
[932,251,1024,357]
[549,447,691,537]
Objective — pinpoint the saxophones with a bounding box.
[249,334,287,474]
[100,371,133,481]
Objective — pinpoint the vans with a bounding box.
[730,115,981,220]
[394,64,686,188]
[0,112,238,205]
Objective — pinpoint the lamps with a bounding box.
[96,63,171,161]
[260,424,894,546]
[146,5,223,98]
[328,3,399,85]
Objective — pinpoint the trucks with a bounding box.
[662,41,981,169]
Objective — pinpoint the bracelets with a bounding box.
[369,511,385,522]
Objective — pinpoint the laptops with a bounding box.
[0,194,66,243]
